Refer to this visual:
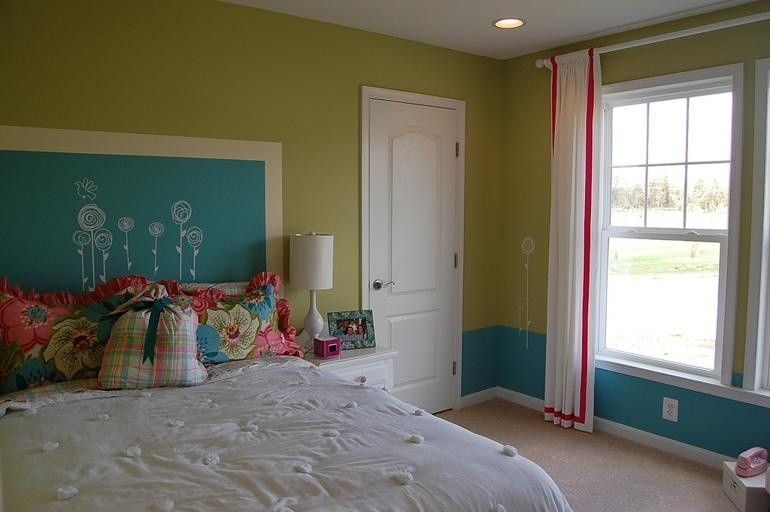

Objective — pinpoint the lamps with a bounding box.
[289,233,333,349]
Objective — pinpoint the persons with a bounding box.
[337,319,367,340]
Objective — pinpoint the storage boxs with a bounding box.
[723,461,770,512]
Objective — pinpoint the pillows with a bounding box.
[0,275,145,395]
[182,281,248,295]
[97,284,207,388]
[155,272,304,362]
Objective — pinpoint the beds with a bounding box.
[0,124,572,512]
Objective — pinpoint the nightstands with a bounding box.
[305,346,398,395]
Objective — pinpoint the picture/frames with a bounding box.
[328,310,376,349]
